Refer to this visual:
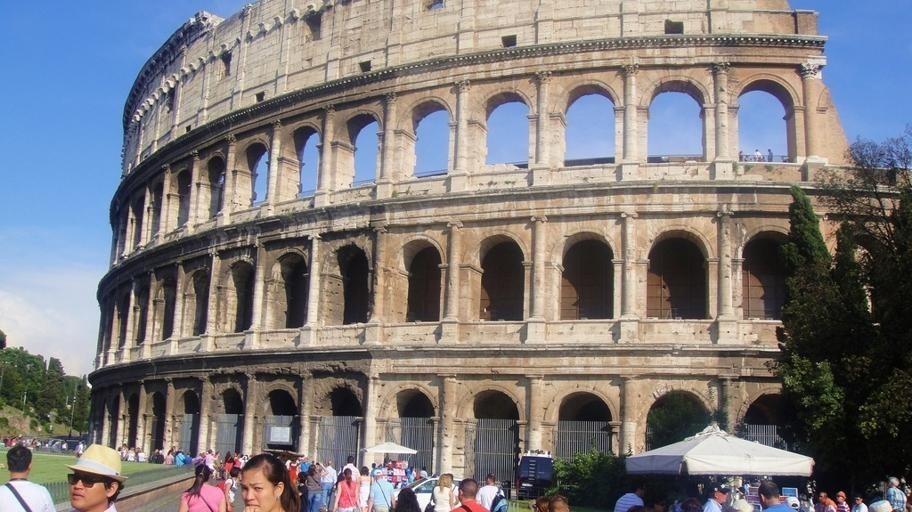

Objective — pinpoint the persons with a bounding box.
[116,443,149,462]
[535,495,568,511]
[614,480,754,511]
[1,445,56,511]
[149,446,338,511]
[744,477,911,512]
[64,444,127,511]
[4,437,87,456]
[332,453,504,511]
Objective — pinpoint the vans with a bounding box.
[513,450,559,503]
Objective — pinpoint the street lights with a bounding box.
[65,395,76,438]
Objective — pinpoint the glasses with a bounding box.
[67,473,107,488]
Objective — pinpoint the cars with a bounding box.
[392,473,464,512]
[1,435,84,458]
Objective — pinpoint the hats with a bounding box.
[371,469,383,476]
[869,500,894,512]
[889,477,900,486]
[65,444,128,483]
[710,482,731,494]
[786,497,801,509]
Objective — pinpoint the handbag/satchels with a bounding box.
[490,494,509,512]
